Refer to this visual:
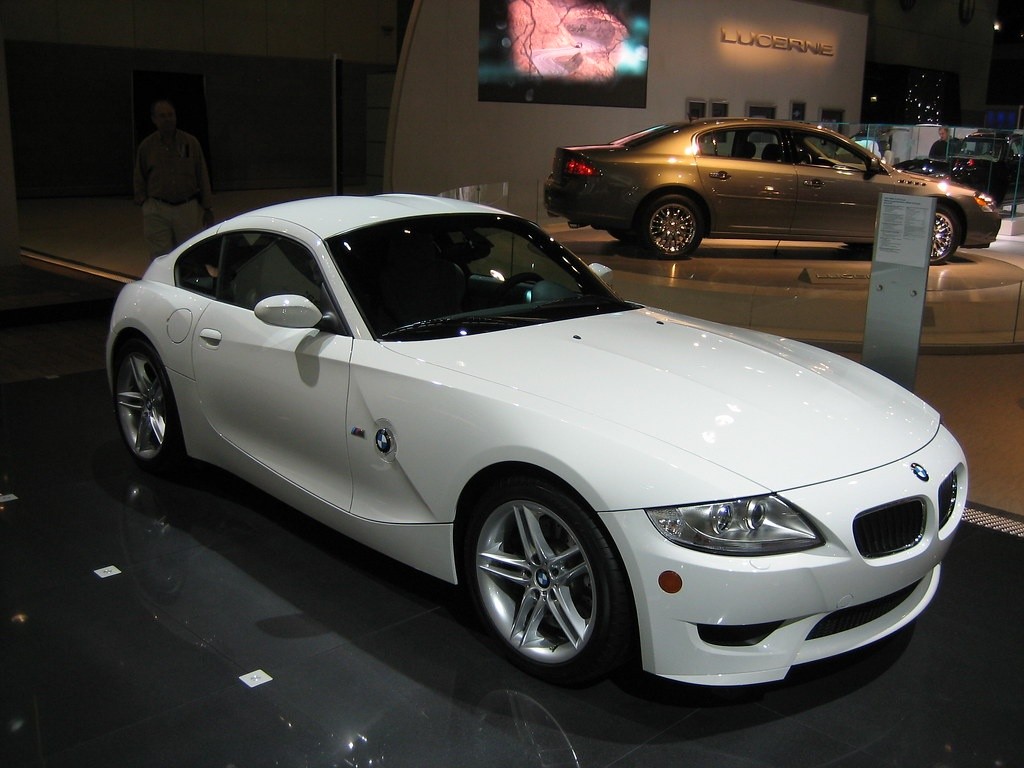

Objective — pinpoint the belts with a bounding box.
[150,193,197,206]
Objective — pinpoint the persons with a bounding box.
[928,126,961,161]
[131,99,216,260]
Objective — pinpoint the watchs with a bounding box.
[204,207,215,212]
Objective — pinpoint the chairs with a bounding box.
[761,143,784,162]
[254,253,314,298]
[372,234,466,325]
[736,141,756,159]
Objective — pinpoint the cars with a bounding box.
[891,157,1006,204]
[946,129,1024,182]
[541,115,1005,265]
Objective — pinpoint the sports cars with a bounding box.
[104,192,972,688]
